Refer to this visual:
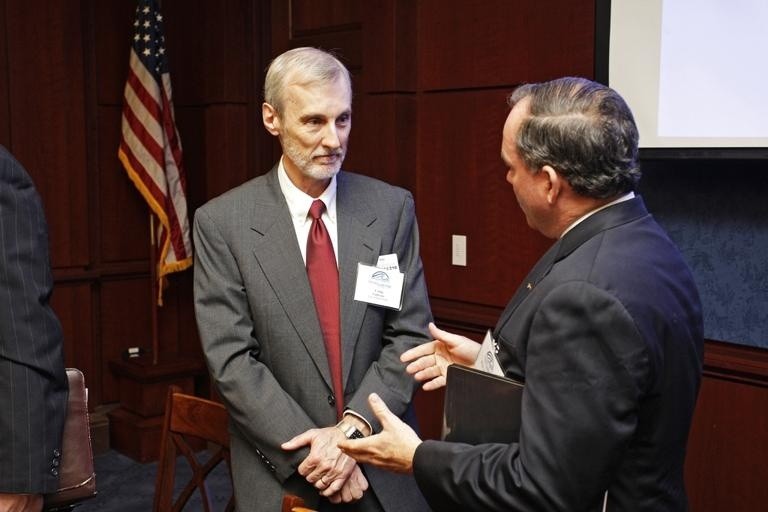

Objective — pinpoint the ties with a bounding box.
[305,199,344,426]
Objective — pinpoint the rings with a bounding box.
[320,476,328,487]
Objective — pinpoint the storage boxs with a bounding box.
[119,363,175,414]
[107,407,185,464]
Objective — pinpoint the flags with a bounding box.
[118,2,199,304]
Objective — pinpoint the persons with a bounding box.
[0,142,72,509]
[192,47,438,512]
[336,73,707,510]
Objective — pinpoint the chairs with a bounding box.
[152,383,238,512]
[281,493,320,512]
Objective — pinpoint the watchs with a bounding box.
[335,417,364,439]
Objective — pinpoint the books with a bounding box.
[441,363,528,450]
